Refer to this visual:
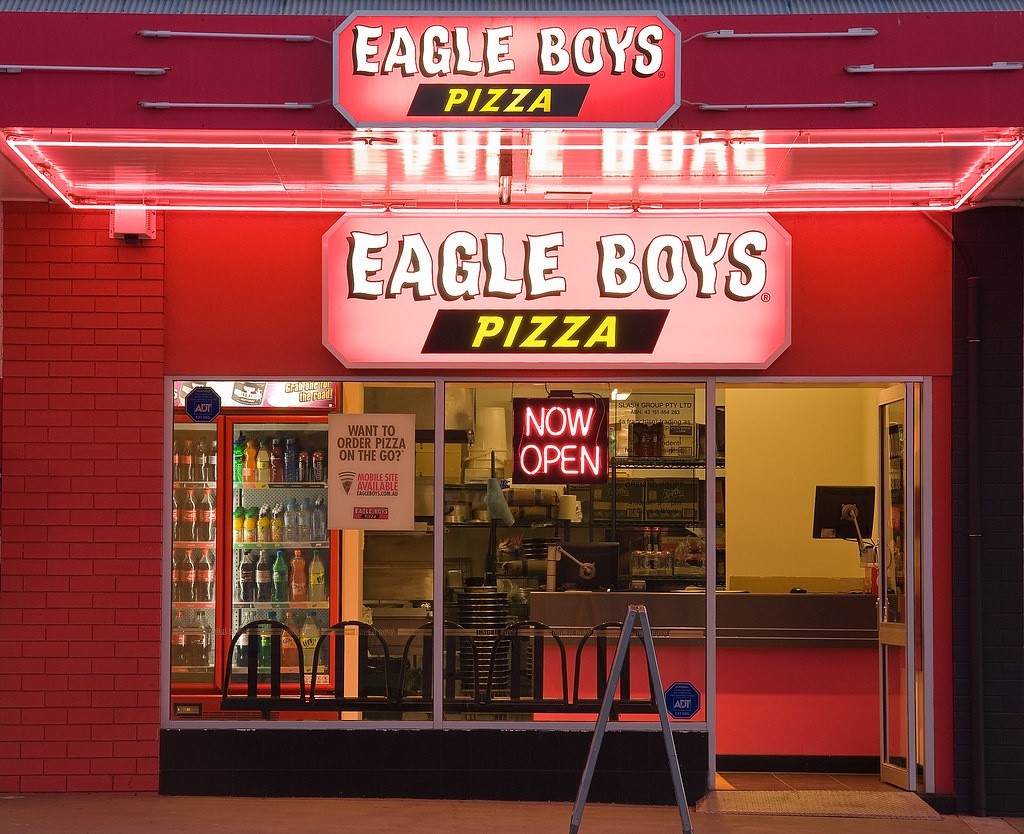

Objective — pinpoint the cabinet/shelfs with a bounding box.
[447,457,726,589]
[362,522,435,619]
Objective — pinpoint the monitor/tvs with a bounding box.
[556,541,619,584]
[813,485,875,539]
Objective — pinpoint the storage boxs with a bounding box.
[673,550,706,577]
[645,478,701,521]
[630,551,673,577]
[564,477,645,522]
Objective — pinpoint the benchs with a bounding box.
[220,620,660,722]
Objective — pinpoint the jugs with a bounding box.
[444,501,470,521]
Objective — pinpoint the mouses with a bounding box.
[790,586,806,593]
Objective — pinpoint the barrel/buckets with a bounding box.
[462,407,509,469]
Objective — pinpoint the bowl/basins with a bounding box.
[474,509,490,521]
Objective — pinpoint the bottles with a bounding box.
[171,438,217,667]
[234,434,326,667]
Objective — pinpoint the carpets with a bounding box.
[696,789,945,822]
